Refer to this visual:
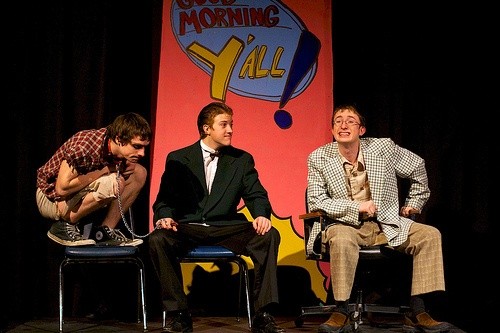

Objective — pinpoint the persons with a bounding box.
[307,106,453,333]
[144,102,284,333]
[35,113,150,247]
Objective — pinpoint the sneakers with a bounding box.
[403,310,450,333]
[95,225,143,246]
[318,311,353,333]
[47,219,96,246]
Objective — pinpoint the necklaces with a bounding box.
[107,137,125,166]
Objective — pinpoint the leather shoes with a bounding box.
[252,312,286,333]
[159,311,193,333]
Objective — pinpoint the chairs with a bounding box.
[154,246,254,330]
[58,215,148,330]
[295,186,412,333]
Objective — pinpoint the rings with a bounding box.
[161,219,164,221]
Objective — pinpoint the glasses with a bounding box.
[333,119,361,126]
